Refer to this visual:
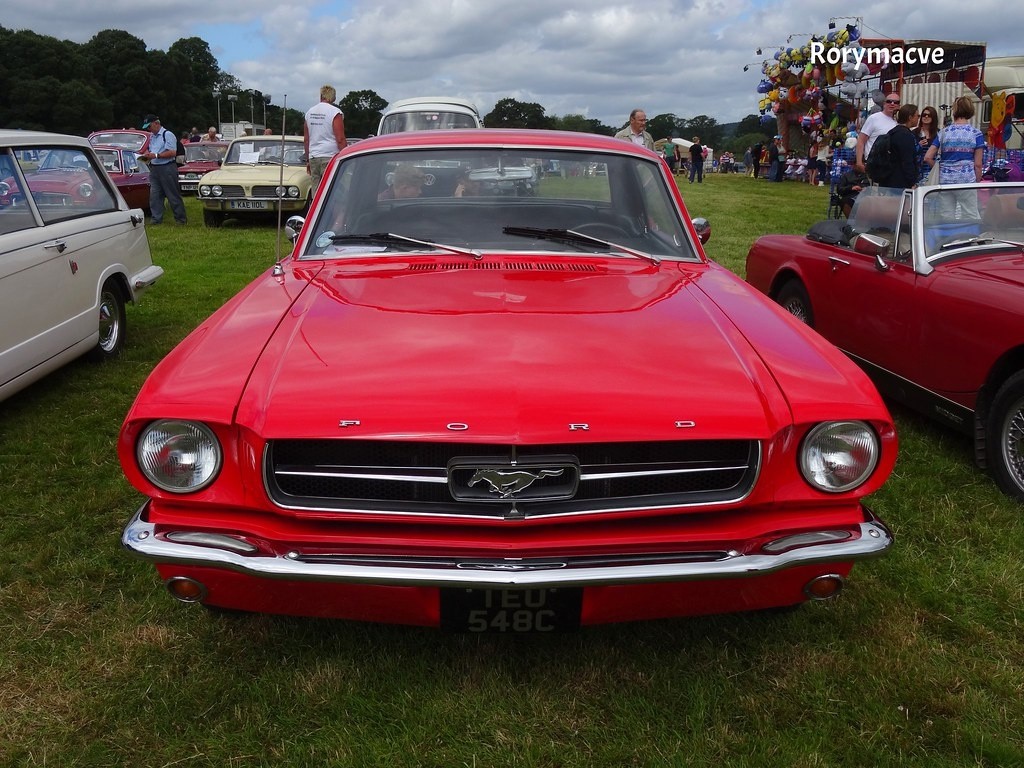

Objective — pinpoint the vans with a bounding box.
[903,56,1024,180]
[341,96,536,198]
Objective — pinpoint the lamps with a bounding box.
[939,104,948,110]
[743,16,864,73]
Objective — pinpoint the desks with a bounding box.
[677,168,687,178]
[759,165,771,179]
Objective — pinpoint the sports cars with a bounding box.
[116,128,899,634]
[745,181,1024,503]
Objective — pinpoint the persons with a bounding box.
[912,105,941,189]
[142,114,188,225]
[264,129,272,135]
[199,126,220,142]
[615,108,656,152]
[838,155,870,221]
[809,138,833,186]
[744,142,766,179]
[451,162,484,197]
[305,85,347,199]
[662,136,680,177]
[767,135,787,182]
[180,127,201,144]
[856,93,901,196]
[540,158,550,180]
[924,95,987,221]
[720,152,735,173]
[378,164,426,200]
[688,137,705,184]
[877,104,921,196]
[830,129,844,149]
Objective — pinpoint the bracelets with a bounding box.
[155,154,158,158]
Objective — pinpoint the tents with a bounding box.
[654,138,713,173]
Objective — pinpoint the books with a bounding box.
[135,154,151,163]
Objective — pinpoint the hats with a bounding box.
[142,114,159,129]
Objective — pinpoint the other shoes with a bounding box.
[818,181,824,186]
[809,182,814,184]
[689,181,692,183]
[698,181,701,183]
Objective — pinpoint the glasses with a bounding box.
[922,113,931,118]
[885,100,900,105]
[778,139,779,140]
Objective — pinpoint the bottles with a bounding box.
[921,131,926,140]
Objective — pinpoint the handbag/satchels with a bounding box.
[163,129,185,156]
[926,160,939,199]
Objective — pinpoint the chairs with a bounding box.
[114,160,130,171]
[369,204,470,241]
[191,151,205,160]
[476,206,602,243]
[208,150,219,159]
[983,192,1024,244]
[853,195,933,258]
[828,175,844,219]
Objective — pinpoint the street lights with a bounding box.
[228,95,238,124]
[212,91,222,133]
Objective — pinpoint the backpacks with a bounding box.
[865,133,891,183]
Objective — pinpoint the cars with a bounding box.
[177,141,231,195]
[0,127,156,218]
[0,129,162,402]
[196,134,315,227]
